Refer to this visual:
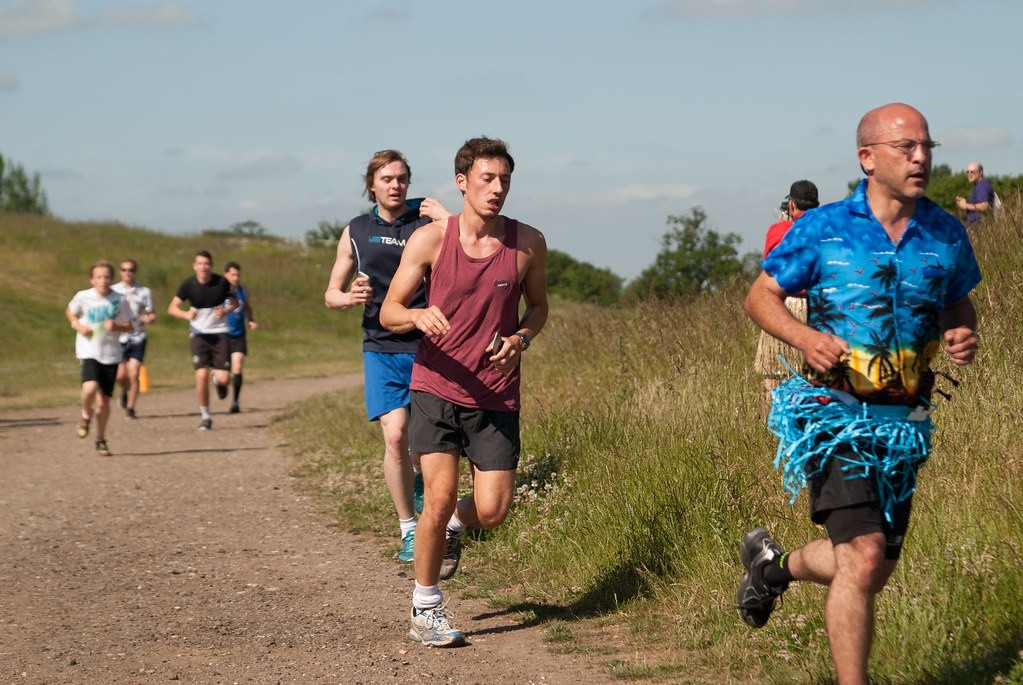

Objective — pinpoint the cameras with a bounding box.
[780,198,791,220]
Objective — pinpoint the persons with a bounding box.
[224,261,259,412]
[753,180,820,390]
[167,250,240,431]
[325,149,454,563]
[110,259,157,419]
[737,103,983,685]
[65,262,136,456]
[957,162,996,224]
[379,138,548,646]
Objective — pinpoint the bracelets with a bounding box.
[70,320,77,329]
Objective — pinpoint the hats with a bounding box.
[785,180,818,200]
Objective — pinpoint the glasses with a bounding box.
[864,139,942,153]
[966,171,975,173]
[121,269,134,272]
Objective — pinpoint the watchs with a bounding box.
[514,332,531,349]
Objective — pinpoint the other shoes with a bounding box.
[216,386,228,399]
[126,407,136,419]
[199,419,212,430]
[95,439,110,455]
[119,391,127,409]
[78,417,91,438]
[230,405,239,414]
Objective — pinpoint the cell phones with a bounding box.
[492,331,503,356]
[357,271,370,294]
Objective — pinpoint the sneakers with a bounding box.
[409,594,466,648]
[736,525,785,629]
[437,528,462,580]
[398,529,416,562]
[412,471,424,514]
